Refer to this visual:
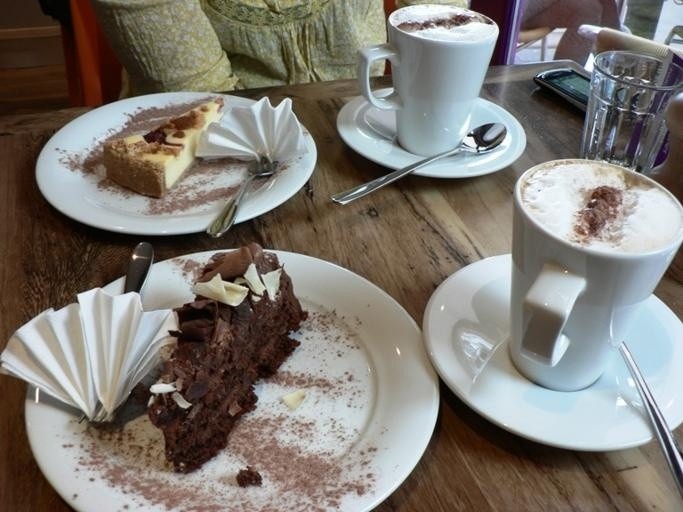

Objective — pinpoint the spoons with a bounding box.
[331,122,509,209]
[205,157,279,240]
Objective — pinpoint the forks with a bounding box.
[74,242,156,427]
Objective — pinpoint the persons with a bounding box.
[521,0,634,69]
[97,1,472,95]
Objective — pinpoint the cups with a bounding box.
[506,156,683,391]
[356,2,500,158]
[578,48,683,179]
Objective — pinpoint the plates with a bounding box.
[23,247,443,512]
[421,250,683,455]
[335,86,528,181]
[32,90,318,239]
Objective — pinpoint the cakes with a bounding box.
[148,242,307,475]
[99,95,226,200]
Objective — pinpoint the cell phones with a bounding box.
[532,67,591,113]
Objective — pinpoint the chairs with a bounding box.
[70,1,122,107]
